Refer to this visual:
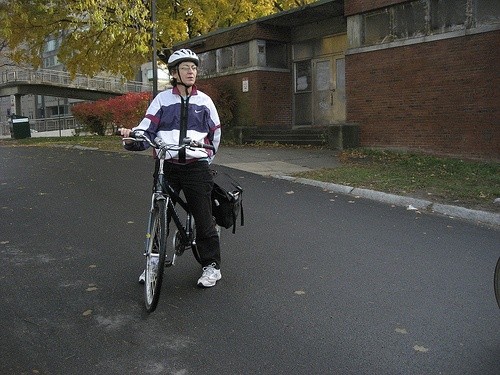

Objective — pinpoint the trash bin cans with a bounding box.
[7,115,32,139]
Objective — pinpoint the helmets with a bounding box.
[166,48,200,68]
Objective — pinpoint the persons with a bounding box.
[118,48,221,287]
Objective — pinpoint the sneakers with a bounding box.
[137,257,159,284]
[196,262,222,287]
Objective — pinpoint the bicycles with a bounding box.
[115,128,221,313]
